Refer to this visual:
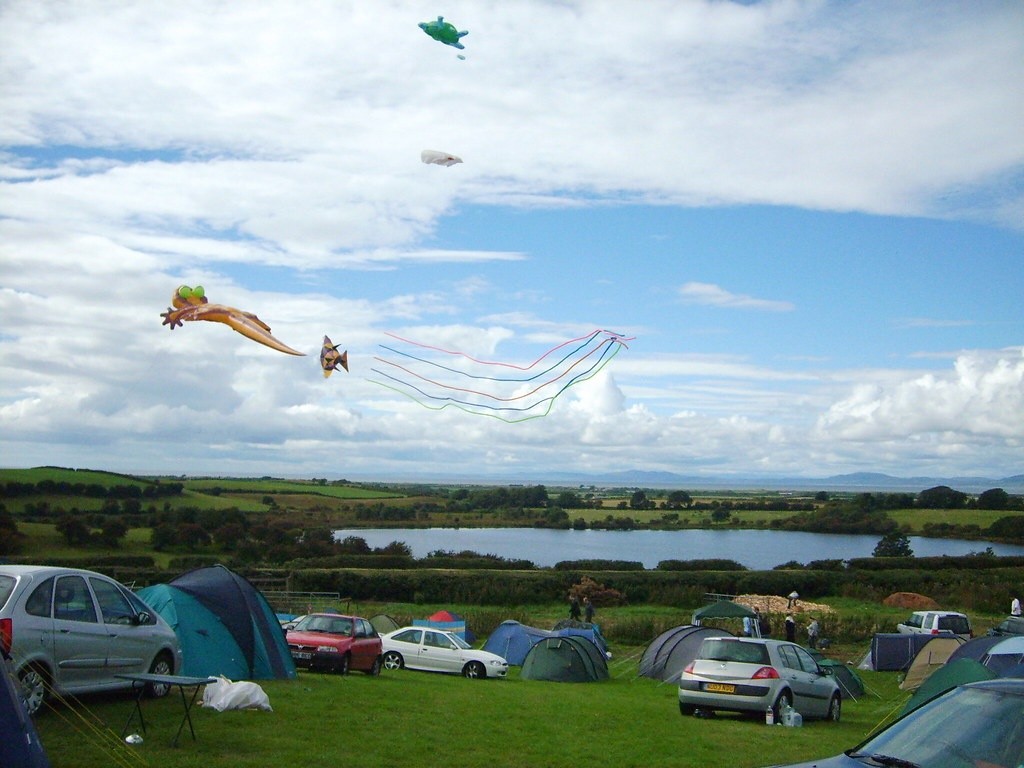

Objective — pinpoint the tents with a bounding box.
[368,614,401,634]
[0,649,52,768]
[898,635,1024,717]
[817,659,866,699]
[691,600,757,638]
[872,632,959,672]
[639,624,736,684]
[482,619,610,682]
[807,648,827,661]
[413,610,477,644]
[856,651,873,670]
[117,584,250,680]
[167,564,299,680]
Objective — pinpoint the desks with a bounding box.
[111,673,217,747]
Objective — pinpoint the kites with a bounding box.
[160,284,307,357]
[419,16,468,49]
[320,335,348,379]
[365,329,637,424]
[421,149,464,167]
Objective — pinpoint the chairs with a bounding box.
[50,583,77,620]
[433,636,440,646]
[425,635,432,646]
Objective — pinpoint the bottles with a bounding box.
[766,706,773,724]
[782,705,802,727]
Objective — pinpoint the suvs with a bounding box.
[986,615,1024,637]
[896,610,974,642]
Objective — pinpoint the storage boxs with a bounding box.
[786,712,802,727]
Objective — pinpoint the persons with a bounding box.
[583,598,595,623]
[743,616,752,637]
[1008,594,1021,617]
[786,611,796,643]
[568,595,582,622]
[788,591,798,609]
[752,606,770,639]
[807,617,818,649]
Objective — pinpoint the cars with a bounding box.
[281,614,308,630]
[676,636,842,723]
[378,625,509,680]
[770,677,1024,768]
[285,612,383,677]
[0,563,181,719]
